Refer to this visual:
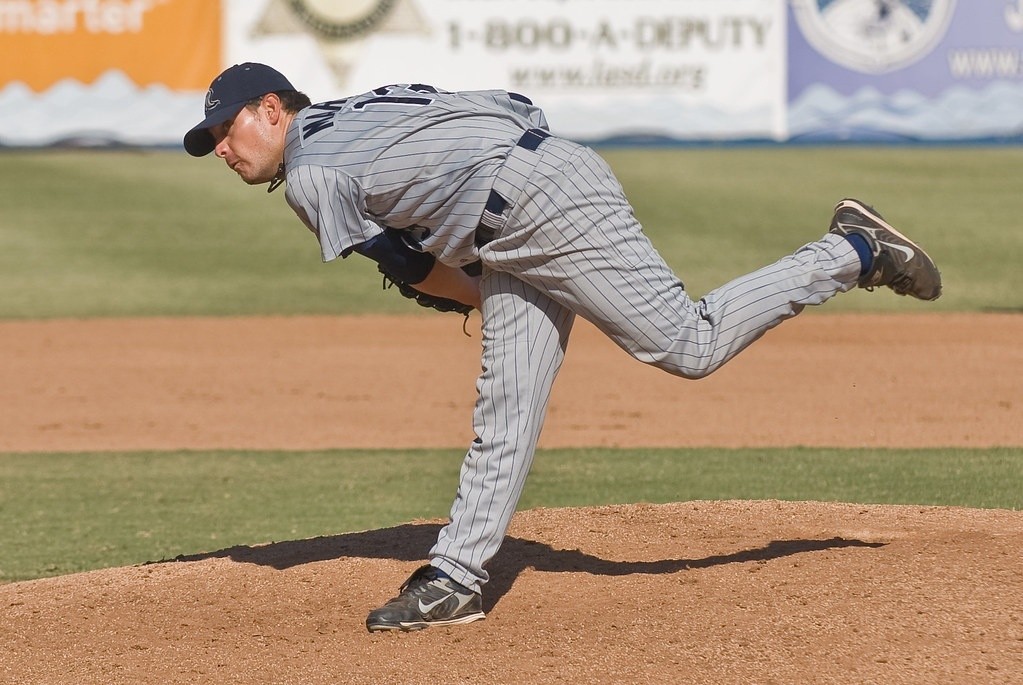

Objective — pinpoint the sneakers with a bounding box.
[829,199,943,304]
[365,563,486,634]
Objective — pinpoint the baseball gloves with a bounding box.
[375,261,484,314]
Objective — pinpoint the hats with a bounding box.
[183,61,298,158]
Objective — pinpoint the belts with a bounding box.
[475,129,549,243]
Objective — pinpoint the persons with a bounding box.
[184,61,942,632]
